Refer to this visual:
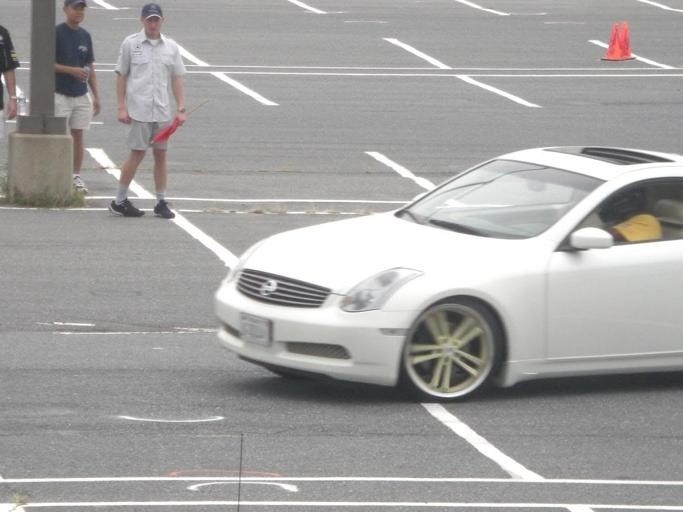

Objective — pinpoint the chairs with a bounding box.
[655,199,683,238]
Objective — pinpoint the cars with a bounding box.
[214,144,682,405]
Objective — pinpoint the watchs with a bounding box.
[177,107,185,113]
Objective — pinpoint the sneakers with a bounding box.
[154,199,178,218]
[73,174,90,195]
[108,198,145,217]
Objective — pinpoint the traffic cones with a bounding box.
[600,23,623,61]
[619,21,635,60]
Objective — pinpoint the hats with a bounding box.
[141,4,163,21]
[63,0,88,6]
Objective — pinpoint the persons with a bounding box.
[0,25,20,121]
[55,0,100,193]
[108,3,187,218]
[601,189,663,242]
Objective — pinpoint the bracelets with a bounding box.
[9,96,17,100]
